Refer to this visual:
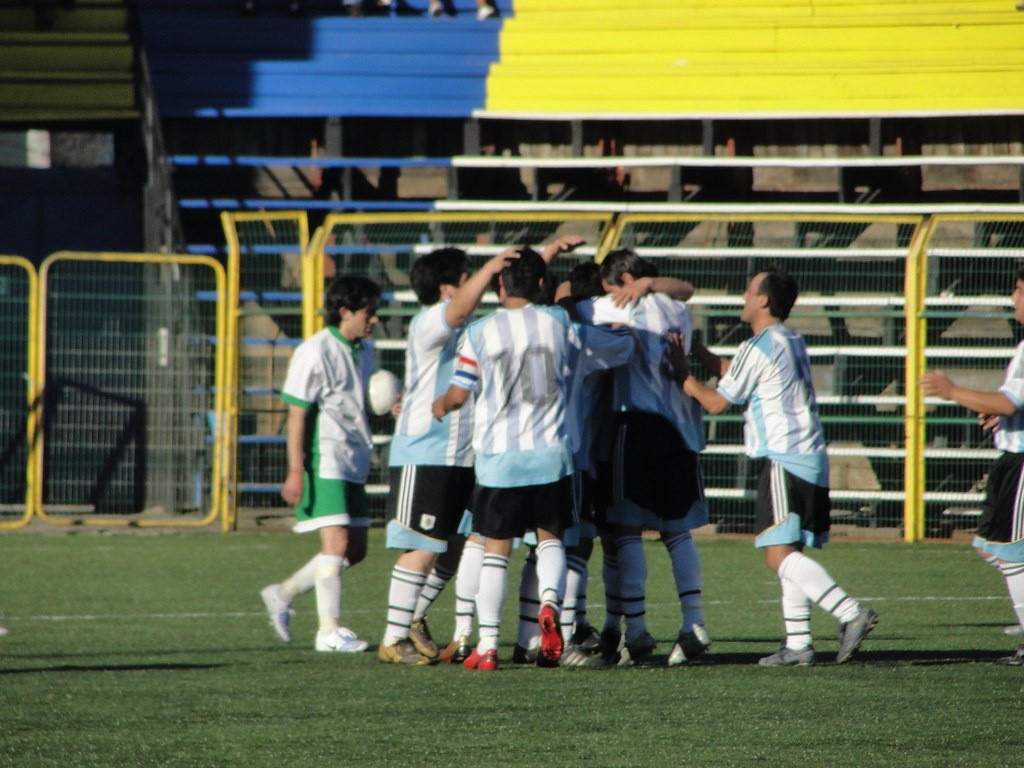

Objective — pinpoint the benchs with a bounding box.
[1,0,1024,531]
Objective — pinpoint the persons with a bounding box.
[378,235,711,670]
[916,274,1024,666]
[242,0,497,21]
[664,272,879,668]
[260,275,383,652]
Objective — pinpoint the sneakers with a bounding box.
[440,637,470,664]
[513,624,622,670]
[261,584,294,642]
[408,617,439,659]
[464,649,496,671]
[756,644,818,669]
[537,605,564,668]
[315,624,371,655]
[838,608,879,666]
[378,638,431,665]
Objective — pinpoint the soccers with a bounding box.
[365,368,399,417]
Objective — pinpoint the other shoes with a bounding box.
[667,623,710,666]
[618,631,659,665]
[996,645,1024,665]
[1004,624,1023,635]
